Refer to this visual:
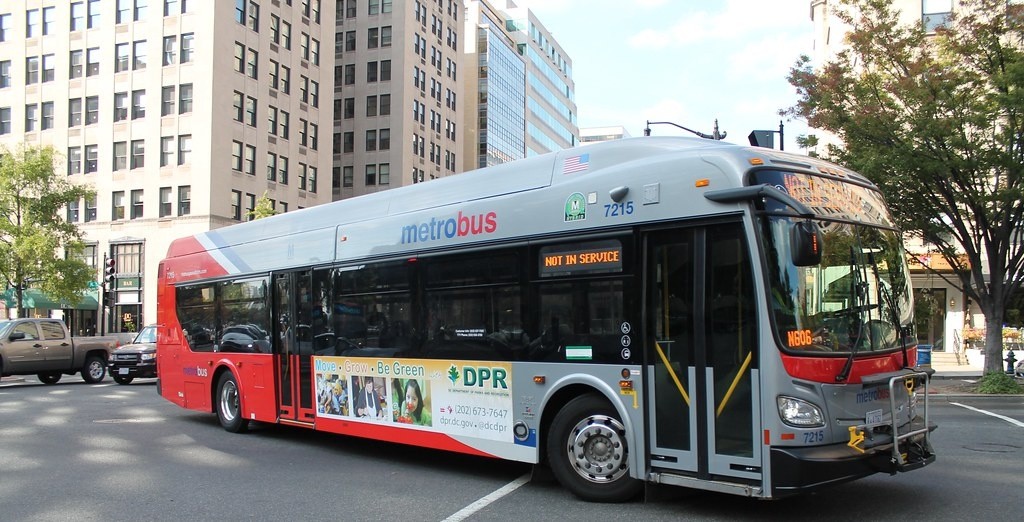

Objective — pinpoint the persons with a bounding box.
[391,378,404,422]
[400,379,431,426]
[770,266,834,352]
[316,373,348,416]
[355,377,383,418]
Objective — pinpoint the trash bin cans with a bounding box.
[916,344,934,368]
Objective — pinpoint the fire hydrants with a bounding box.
[1006,350,1016,375]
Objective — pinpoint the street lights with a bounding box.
[644,118,727,140]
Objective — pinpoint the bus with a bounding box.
[156,135,938,505]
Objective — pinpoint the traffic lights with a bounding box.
[105,258,115,282]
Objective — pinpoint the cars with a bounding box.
[189,323,272,353]
[108,323,157,385]
[314,333,359,356]
[1016,360,1024,378]
[269,325,313,356]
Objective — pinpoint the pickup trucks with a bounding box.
[0,318,121,384]
